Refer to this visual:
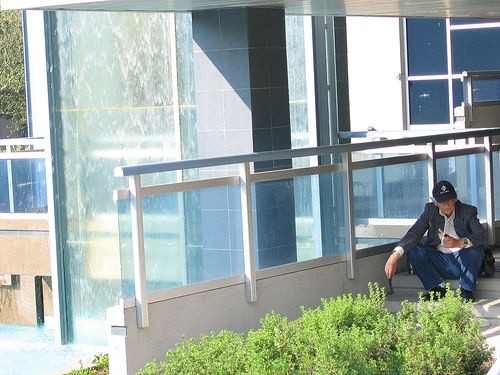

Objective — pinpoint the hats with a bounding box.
[432,180,458,203]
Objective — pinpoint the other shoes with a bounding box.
[419,286,448,303]
[458,288,474,302]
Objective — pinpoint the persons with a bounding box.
[384,180,487,303]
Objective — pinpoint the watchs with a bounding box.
[460,238,468,248]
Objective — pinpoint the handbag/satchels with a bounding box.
[482,248,496,276]
